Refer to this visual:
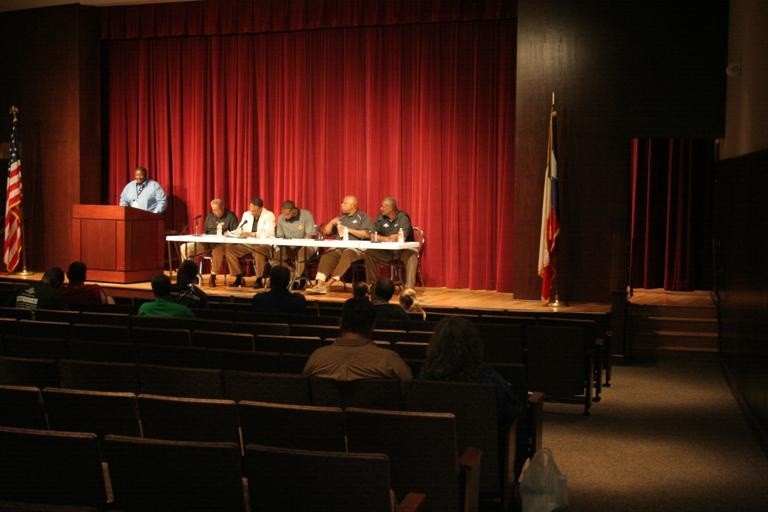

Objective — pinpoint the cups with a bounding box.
[371,232,382,243]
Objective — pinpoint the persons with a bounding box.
[119,167,168,216]
[137,273,197,319]
[169,260,210,309]
[414,314,524,423]
[60,260,116,305]
[267,199,318,291]
[223,197,277,289]
[399,286,426,319]
[364,196,419,292]
[179,198,240,288]
[305,194,375,295]
[14,266,68,321]
[250,265,306,308]
[369,278,409,320]
[300,296,414,382]
[350,280,369,297]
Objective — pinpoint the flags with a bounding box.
[2,119,23,273]
[536,108,562,307]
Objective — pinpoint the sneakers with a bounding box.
[400,288,417,297]
[293,278,301,290]
[305,281,330,294]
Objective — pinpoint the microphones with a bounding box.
[128,198,136,205]
[192,213,202,220]
[239,220,248,229]
[315,222,325,232]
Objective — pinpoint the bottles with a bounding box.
[217,223,222,237]
[343,226,349,242]
[398,227,404,245]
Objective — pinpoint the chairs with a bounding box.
[390,228,424,291]
[224,372,311,404]
[401,379,542,499]
[345,406,484,512]
[238,401,344,453]
[2,355,32,387]
[429,309,613,410]
[36,359,137,393]
[41,388,141,437]
[99,432,247,511]
[0,385,44,429]
[242,442,425,512]
[135,395,237,444]
[138,294,255,367]
[0,283,137,360]
[312,374,400,406]
[137,363,225,398]
[0,425,105,511]
[256,299,431,376]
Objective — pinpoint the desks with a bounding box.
[166,234,419,294]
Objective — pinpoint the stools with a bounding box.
[166,231,182,281]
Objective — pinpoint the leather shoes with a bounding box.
[230,276,246,287]
[209,276,216,288]
[253,277,263,288]
